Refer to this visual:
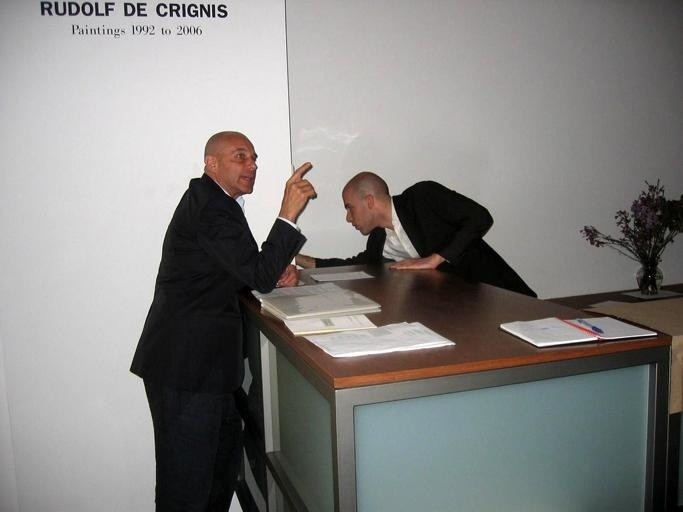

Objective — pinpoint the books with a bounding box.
[250,281,456,357]
[499,315,659,349]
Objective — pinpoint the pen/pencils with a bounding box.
[577,319,603,333]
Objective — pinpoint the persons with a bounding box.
[295,170,538,300]
[129,129,318,512]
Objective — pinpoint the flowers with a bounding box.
[578,176,683,294]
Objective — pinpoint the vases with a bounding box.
[635,266,663,295]
